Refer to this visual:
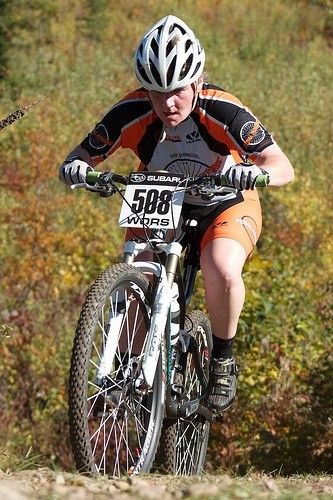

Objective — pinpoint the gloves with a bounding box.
[58,155,93,188]
[225,163,271,192]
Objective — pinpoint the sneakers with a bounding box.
[92,388,128,414]
[206,350,239,410]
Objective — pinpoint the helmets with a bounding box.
[132,14,206,93]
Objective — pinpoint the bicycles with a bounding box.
[57,171,270,481]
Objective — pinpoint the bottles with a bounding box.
[170,282,180,345]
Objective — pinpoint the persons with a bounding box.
[57,14,295,415]
